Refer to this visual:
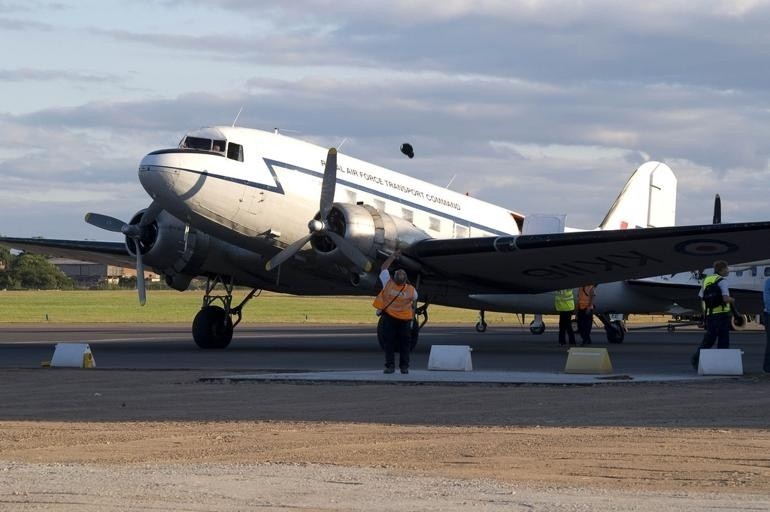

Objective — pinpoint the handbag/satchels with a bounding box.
[703,283,724,309]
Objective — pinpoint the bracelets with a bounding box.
[391,254,395,259]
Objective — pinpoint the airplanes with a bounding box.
[0,127,770,349]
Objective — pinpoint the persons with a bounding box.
[692,260,736,369]
[762,278,770,373]
[555,288,576,347]
[372,250,419,374]
[576,283,599,344]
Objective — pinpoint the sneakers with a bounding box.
[384,367,408,374]
